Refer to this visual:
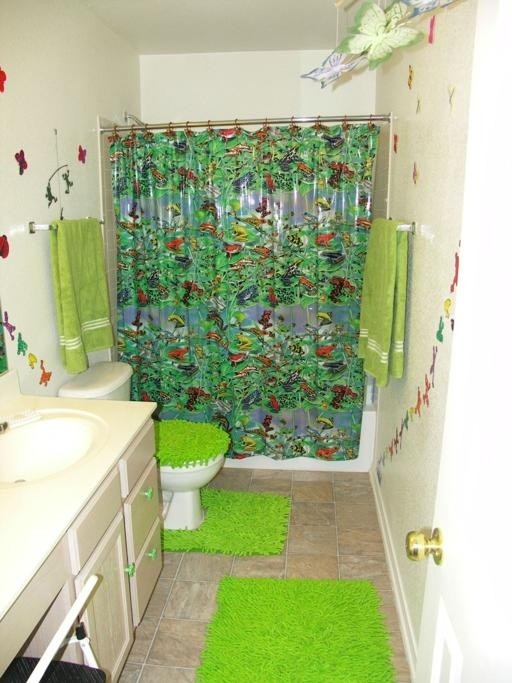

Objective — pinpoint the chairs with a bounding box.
[0,575,110,682]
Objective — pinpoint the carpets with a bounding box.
[195,576,396,683]
[160,487,292,558]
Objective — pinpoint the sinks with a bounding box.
[0,407,109,484]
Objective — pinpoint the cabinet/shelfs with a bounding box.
[121,419,163,629]
[72,465,135,683]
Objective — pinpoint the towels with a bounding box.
[52,217,115,373]
[357,217,412,385]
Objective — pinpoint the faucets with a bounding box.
[0,421,9,432]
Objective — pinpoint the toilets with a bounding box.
[59,361,229,529]
